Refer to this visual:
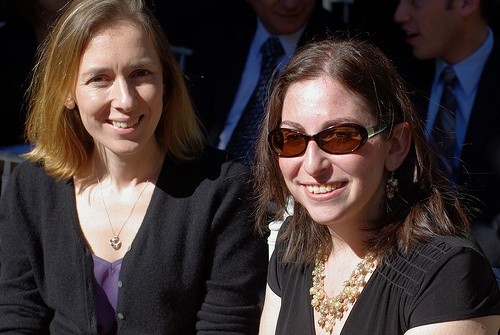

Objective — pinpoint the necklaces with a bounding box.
[309,232,377,332]
[95,155,157,249]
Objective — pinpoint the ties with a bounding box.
[428,65,460,192]
[224,36,286,165]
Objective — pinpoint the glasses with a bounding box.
[267,122,389,158]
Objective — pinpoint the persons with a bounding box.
[394,1,499,271]
[0,0,272,335]
[192,0,352,173]
[253,41,500,335]
